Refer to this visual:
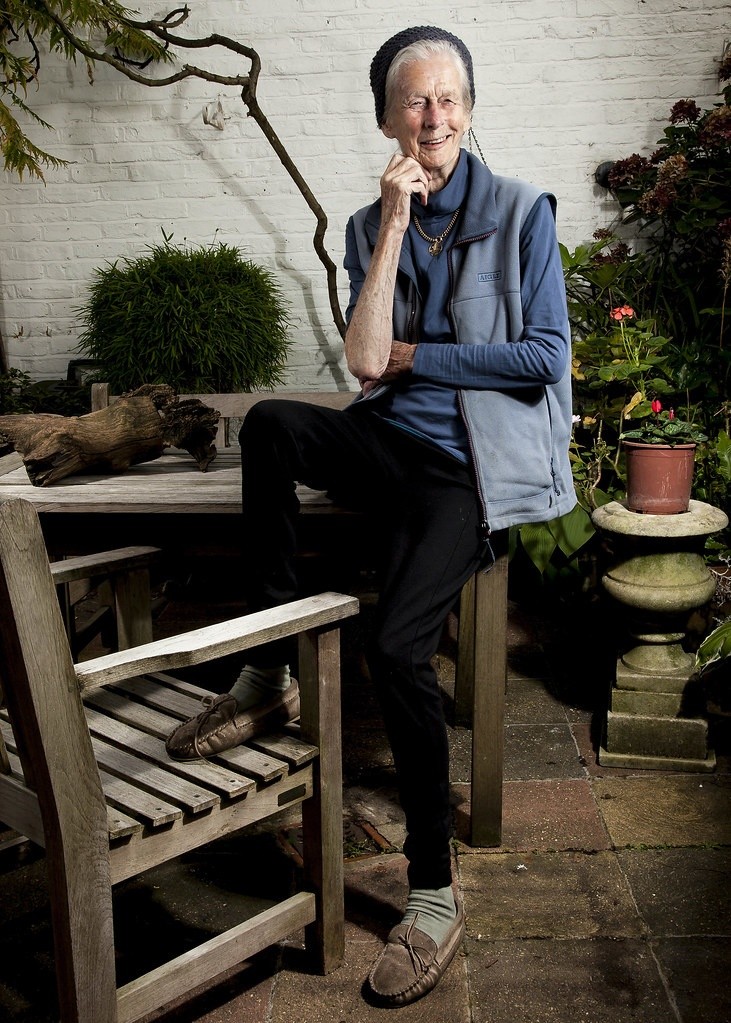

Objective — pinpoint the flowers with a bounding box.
[597,304,706,446]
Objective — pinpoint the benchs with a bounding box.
[90,382,357,452]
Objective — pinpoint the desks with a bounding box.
[1,446,506,848]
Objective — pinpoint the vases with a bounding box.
[622,441,696,515]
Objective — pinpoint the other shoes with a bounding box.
[166,678,301,761]
[367,897,466,1005]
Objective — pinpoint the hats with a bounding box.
[370,25,476,127]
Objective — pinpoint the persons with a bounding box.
[167,26,578,1008]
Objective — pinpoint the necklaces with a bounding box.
[413,209,460,257]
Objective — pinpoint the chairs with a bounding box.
[0,499,360,1023]
